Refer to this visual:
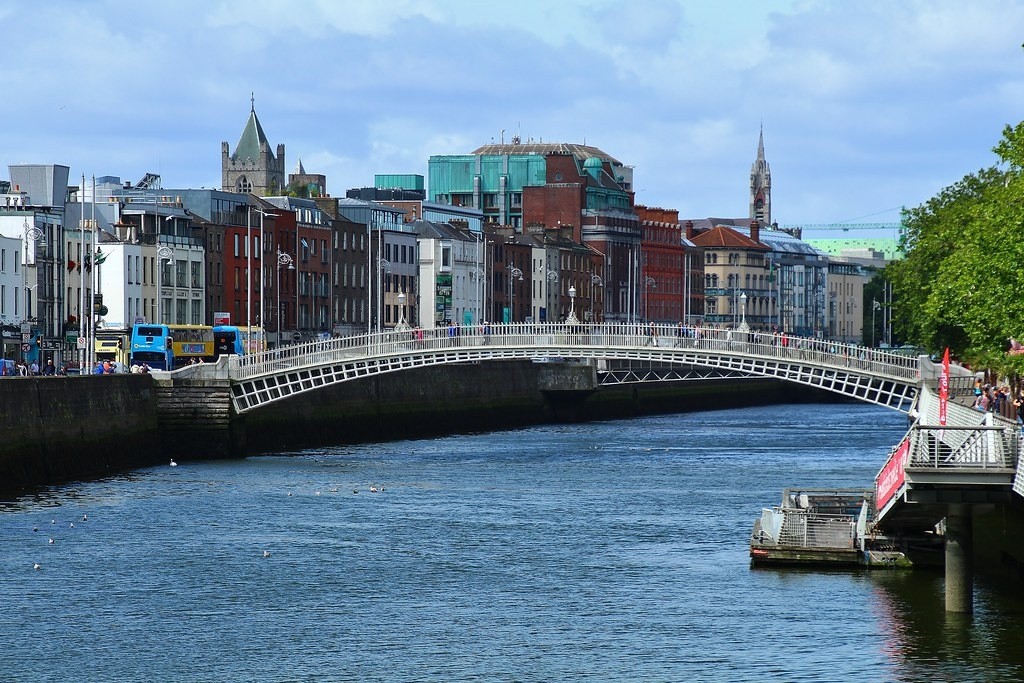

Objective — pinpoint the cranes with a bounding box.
[778,204,914,242]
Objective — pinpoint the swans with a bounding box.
[170,458,177,467]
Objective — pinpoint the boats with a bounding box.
[750,486,877,566]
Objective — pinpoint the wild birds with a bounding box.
[601,446,604,449]
[370,486,378,493]
[49,539,54,543]
[34,563,40,571]
[632,447,651,451]
[354,488,358,494]
[627,444,630,449]
[51,519,55,524]
[381,487,386,492]
[83,515,87,521]
[594,445,600,450]
[69,523,74,528]
[33,527,38,532]
[288,492,292,496]
[287,448,363,463]
[587,446,592,451]
[329,487,341,492]
[262,551,270,558]
[542,447,547,452]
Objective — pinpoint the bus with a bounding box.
[129,323,267,371]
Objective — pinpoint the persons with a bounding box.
[842,347,865,371]
[673,321,702,348]
[478,318,492,346]
[798,338,816,362]
[93,358,117,374]
[1013,387,1024,424]
[129,362,152,373]
[15,359,69,376]
[970,379,1010,415]
[748,329,762,354]
[411,326,424,350]
[950,356,974,371]
[771,331,791,358]
[645,321,659,347]
[190,356,205,365]
[448,322,461,347]
[726,326,735,350]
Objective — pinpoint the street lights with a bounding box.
[155,216,176,324]
[394,291,413,331]
[378,226,392,333]
[590,269,603,323]
[738,291,751,332]
[565,285,581,324]
[872,297,882,348]
[23,204,50,322]
[276,243,295,350]
[510,262,525,324]
[644,272,657,336]
[546,264,558,322]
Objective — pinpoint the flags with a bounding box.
[939,348,950,426]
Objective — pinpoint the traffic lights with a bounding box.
[167,338,172,350]
[36,336,42,347]
[117,337,123,349]
